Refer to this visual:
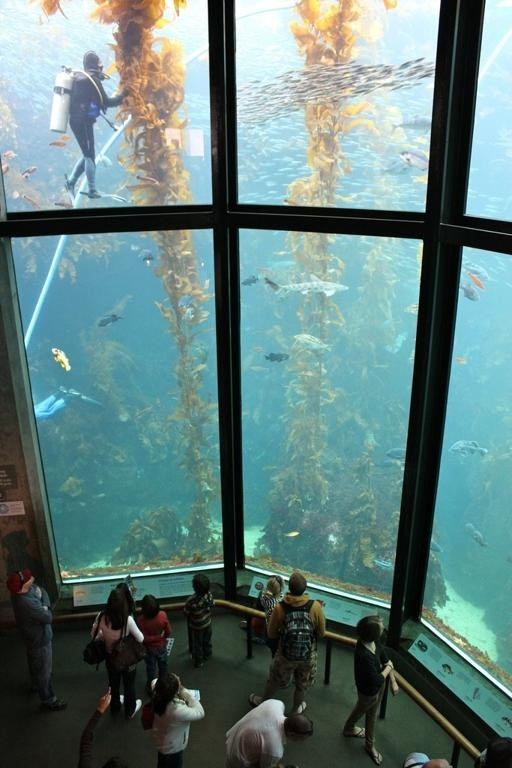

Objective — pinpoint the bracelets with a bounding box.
[385,663,394,670]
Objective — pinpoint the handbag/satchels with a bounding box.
[141,704,154,732]
[247,691,268,707]
[83,637,106,665]
[110,634,149,674]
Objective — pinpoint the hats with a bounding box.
[7,566,31,594]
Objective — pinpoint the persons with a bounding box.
[473,736,512,768]
[63,50,131,200]
[403,751,455,768]
[180,573,216,667]
[150,673,206,768]
[6,566,68,709]
[131,592,175,697]
[238,574,283,647]
[88,587,145,720]
[245,571,327,716]
[340,615,400,764]
[75,684,128,768]
[111,582,145,684]
[222,696,314,768]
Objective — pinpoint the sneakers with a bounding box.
[126,699,142,720]
[110,695,124,710]
[291,701,308,715]
[40,695,68,712]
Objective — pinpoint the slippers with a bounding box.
[343,726,365,737]
[365,745,383,765]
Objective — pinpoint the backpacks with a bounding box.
[278,599,315,662]
